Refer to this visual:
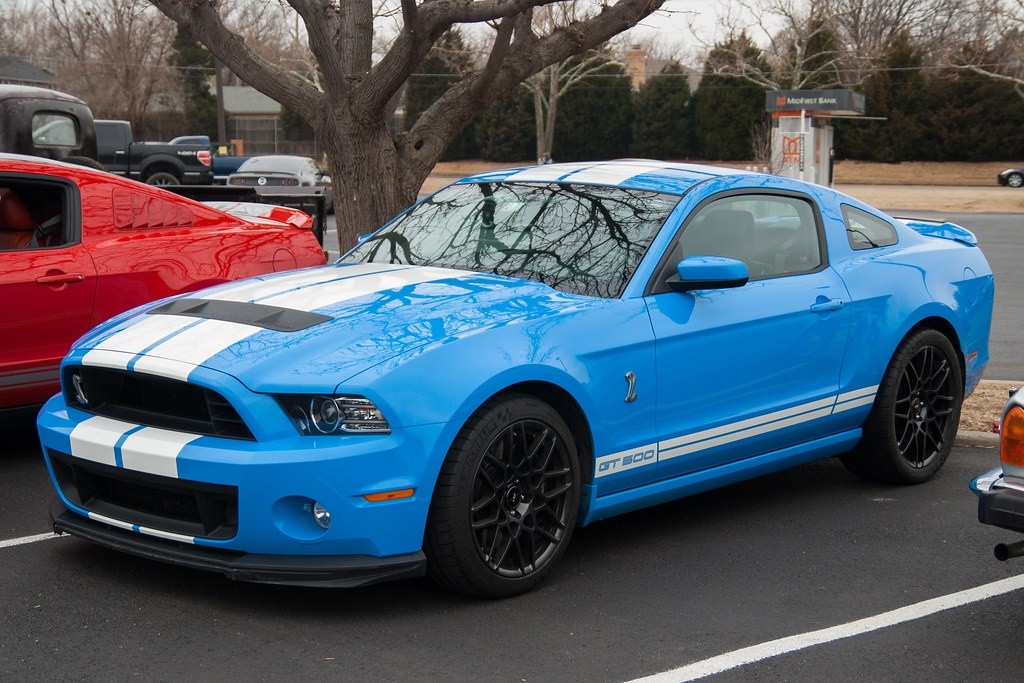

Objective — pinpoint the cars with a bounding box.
[969,383,1024,560]
[226,155,335,215]
[35,157,996,606]
[0,152,330,427]
[997,168,1024,188]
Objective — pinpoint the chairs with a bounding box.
[694,209,773,283]
[0,188,37,250]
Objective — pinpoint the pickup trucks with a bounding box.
[0,83,326,250]
[32,120,215,186]
[168,135,251,185]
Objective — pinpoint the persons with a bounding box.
[538,152,552,165]
